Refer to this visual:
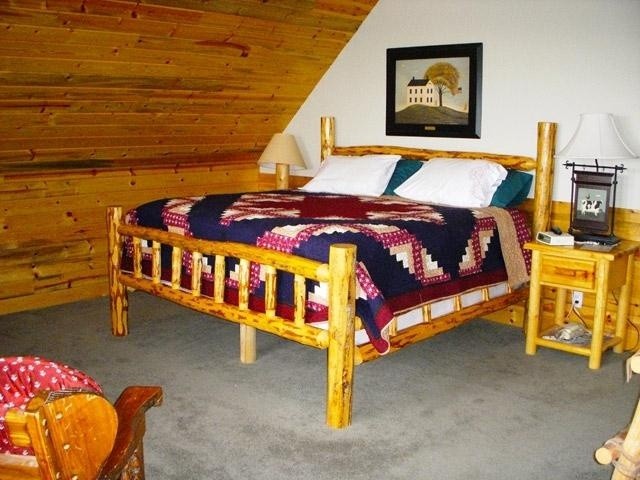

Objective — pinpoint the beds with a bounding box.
[105,117,558,429]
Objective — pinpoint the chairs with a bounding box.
[0,355,162,480]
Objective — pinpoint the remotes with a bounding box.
[551,226,562,235]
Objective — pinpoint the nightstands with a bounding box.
[523,231,640,369]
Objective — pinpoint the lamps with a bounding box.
[553,110,640,245]
[256,133,308,192]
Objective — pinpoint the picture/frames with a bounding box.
[386,42,483,140]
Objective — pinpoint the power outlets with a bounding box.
[573,291,583,309]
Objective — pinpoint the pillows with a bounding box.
[492,168,533,210]
[395,158,507,210]
[384,158,422,199]
[300,153,400,198]
